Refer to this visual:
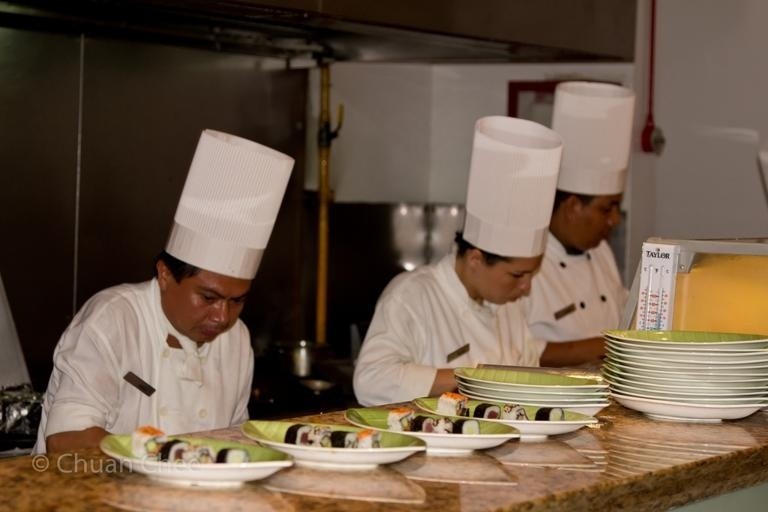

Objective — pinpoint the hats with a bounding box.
[164,127,296,282]
[462,116,563,260]
[551,80,636,197]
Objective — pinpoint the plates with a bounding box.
[95,430,298,490]
[261,464,428,504]
[239,415,427,471]
[558,417,759,481]
[412,394,599,446]
[95,477,294,512]
[394,450,521,488]
[599,329,767,426]
[344,406,519,457]
[452,365,611,418]
[488,439,591,471]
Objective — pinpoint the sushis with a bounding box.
[129,426,247,463]
[437,391,566,422]
[282,422,379,449]
[386,406,479,436]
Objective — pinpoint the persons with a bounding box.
[524,191,633,369]
[351,231,547,407]
[31,253,257,459]
